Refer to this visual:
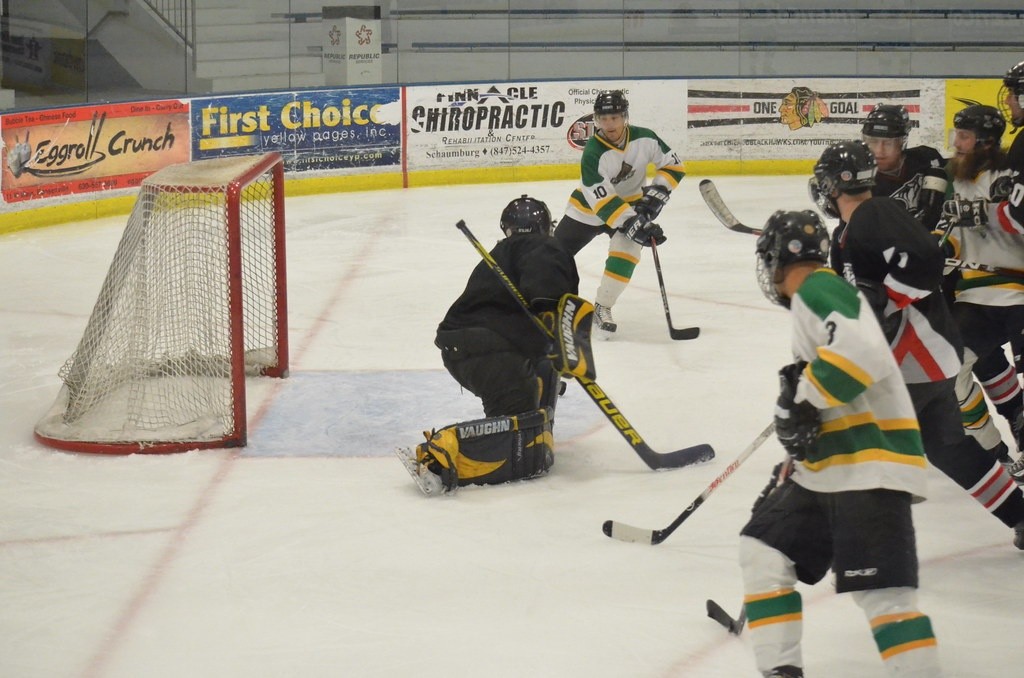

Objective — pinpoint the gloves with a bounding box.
[774,361,825,464]
[991,176,1014,203]
[616,214,667,246]
[627,183,672,220]
[552,332,574,379]
[935,196,988,229]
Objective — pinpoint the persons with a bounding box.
[809,139,1023,554]
[399,195,597,497]
[554,89,686,342]
[737,207,944,678]
[860,60,1024,490]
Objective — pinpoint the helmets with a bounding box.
[814,139,878,194]
[501,198,552,239]
[954,105,1007,137]
[862,101,914,136]
[756,209,832,311]
[594,91,630,116]
[997,61,1024,127]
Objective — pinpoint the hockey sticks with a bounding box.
[939,217,956,251]
[645,213,702,341]
[913,208,925,220]
[704,454,792,636]
[698,177,1024,280]
[455,218,717,471]
[601,420,778,546]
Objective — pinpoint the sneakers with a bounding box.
[395,437,447,498]
[1012,412,1024,453]
[1007,454,1024,488]
[592,302,617,341]
